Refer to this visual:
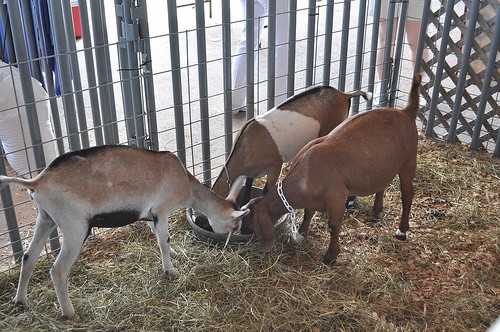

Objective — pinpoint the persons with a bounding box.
[369,0,432,106]
[0,1,72,180]
[231,0,290,113]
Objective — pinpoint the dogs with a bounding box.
[0,145,250,321]
[240,72,422,264]
[211,85,372,209]
